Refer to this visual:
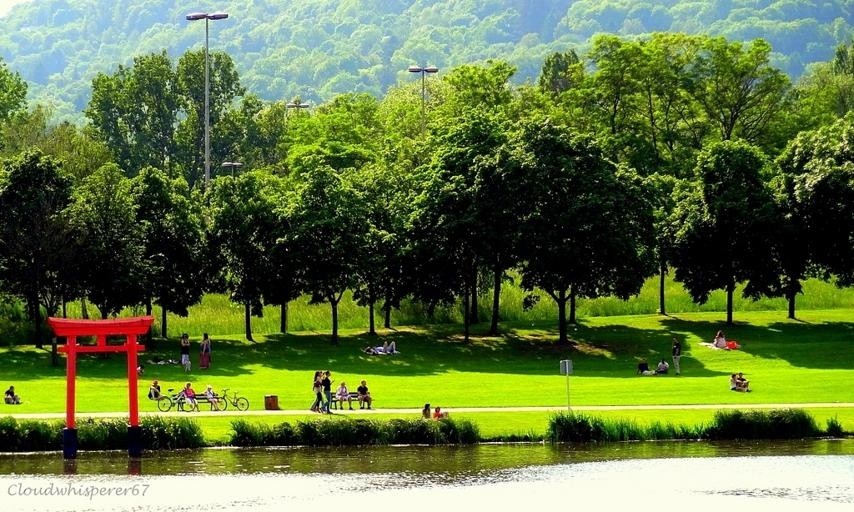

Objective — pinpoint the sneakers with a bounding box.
[310,407,333,414]
[360,407,363,409]
[348,407,353,410]
[339,407,343,410]
[367,407,371,409]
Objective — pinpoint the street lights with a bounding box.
[220,160,244,178]
[407,63,442,138]
[184,11,231,188]
[285,97,310,117]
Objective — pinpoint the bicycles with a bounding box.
[215,388,250,412]
[156,388,193,412]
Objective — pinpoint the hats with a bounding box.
[152,380,158,384]
[207,385,212,388]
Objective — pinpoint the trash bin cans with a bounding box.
[265,396,278,409]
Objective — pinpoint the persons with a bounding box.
[422,404,431,419]
[737,373,749,388]
[714,331,726,349]
[204,385,220,410]
[433,407,443,419]
[5,386,20,405]
[730,374,749,391]
[637,359,657,375]
[309,371,335,414]
[357,381,371,409]
[137,332,211,376]
[362,340,400,355]
[671,336,681,375]
[184,383,200,412]
[148,380,162,401]
[656,358,670,372]
[335,382,353,409]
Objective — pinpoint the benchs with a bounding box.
[171,391,222,412]
[328,390,375,411]
